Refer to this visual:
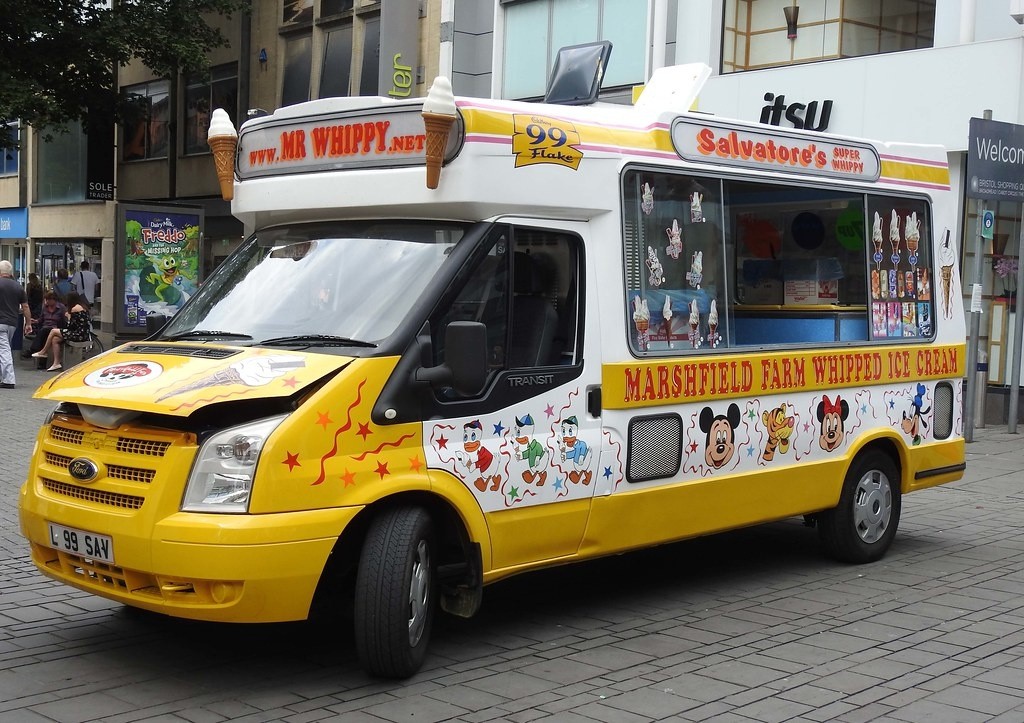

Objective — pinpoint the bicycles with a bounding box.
[81,304,105,360]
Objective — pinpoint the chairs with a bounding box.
[479,253,561,369]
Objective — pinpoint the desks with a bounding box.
[716,304,868,341]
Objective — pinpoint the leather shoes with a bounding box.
[0,382,15,389]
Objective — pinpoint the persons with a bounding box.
[21,261,99,371]
[26,273,43,335]
[0,260,32,389]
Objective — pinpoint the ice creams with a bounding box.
[631,295,718,351]
[155,353,307,403]
[421,75,458,188]
[873,208,921,273]
[207,108,238,201]
[937,227,955,317]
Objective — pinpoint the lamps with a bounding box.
[26,236,31,243]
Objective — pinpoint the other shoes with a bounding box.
[47,364,63,372]
[21,348,32,358]
[36,362,45,369]
[32,353,49,358]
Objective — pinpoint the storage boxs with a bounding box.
[741,256,845,305]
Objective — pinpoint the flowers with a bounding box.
[994,258,1020,297]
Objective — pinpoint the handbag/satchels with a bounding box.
[79,293,92,310]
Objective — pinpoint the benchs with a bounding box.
[25,335,93,372]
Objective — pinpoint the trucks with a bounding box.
[16,38,972,682]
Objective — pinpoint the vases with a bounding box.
[995,294,1016,311]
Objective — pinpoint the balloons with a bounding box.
[791,212,826,249]
[743,219,781,258]
[836,206,865,251]
